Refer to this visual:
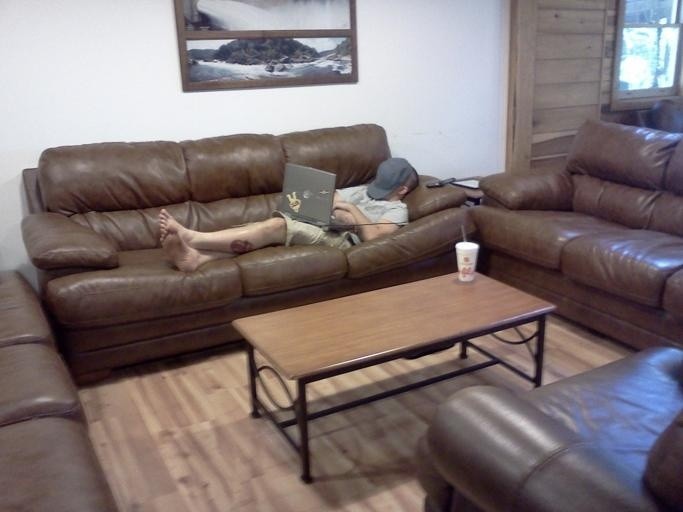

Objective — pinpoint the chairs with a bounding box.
[415,346,682,511]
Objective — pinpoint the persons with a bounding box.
[159,158,419,272]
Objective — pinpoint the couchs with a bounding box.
[17,122,476,384]
[0,268,118,512]
[464,115,683,349]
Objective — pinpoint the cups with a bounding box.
[456,241,480,282]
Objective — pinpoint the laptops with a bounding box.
[277,163,354,231]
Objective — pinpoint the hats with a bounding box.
[366,158,413,200]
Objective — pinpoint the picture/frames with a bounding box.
[174,0,358,94]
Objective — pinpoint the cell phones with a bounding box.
[426,176,455,187]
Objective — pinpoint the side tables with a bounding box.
[456,175,485,206]
